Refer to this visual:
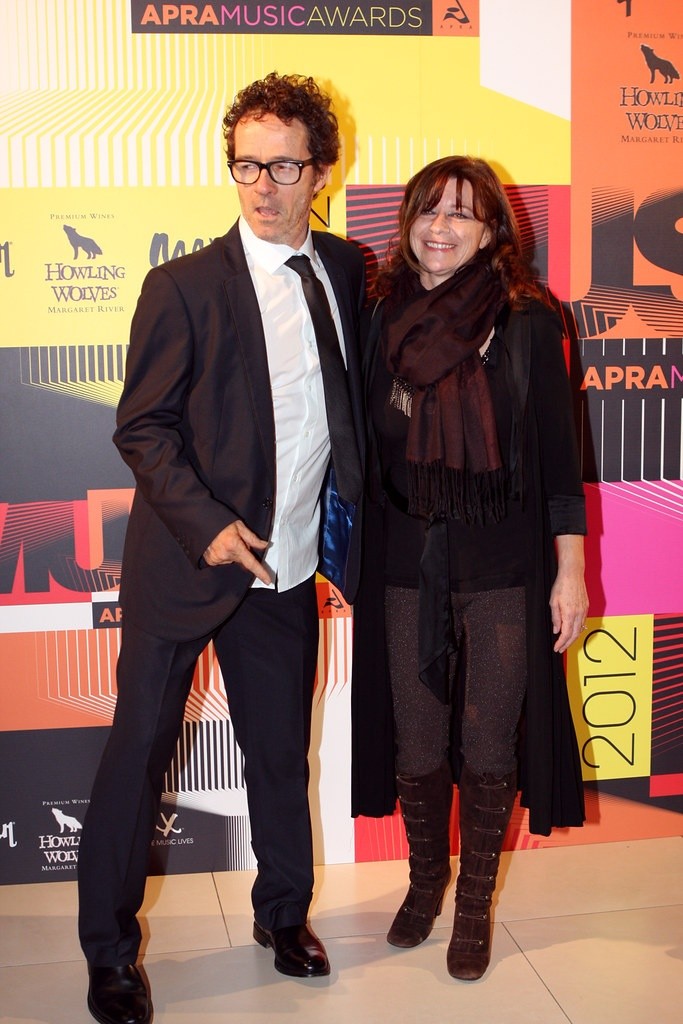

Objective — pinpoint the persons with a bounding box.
[359,157,590,983]
[78,73,368,1023]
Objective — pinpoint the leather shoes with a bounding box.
[87,961,150,1024]
[253,919,330,977]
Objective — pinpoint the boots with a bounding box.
[447,762,517,980]
[387,758,454,948]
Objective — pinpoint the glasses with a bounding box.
[227,157,314,185]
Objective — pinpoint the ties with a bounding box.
[284,254,364,507]
